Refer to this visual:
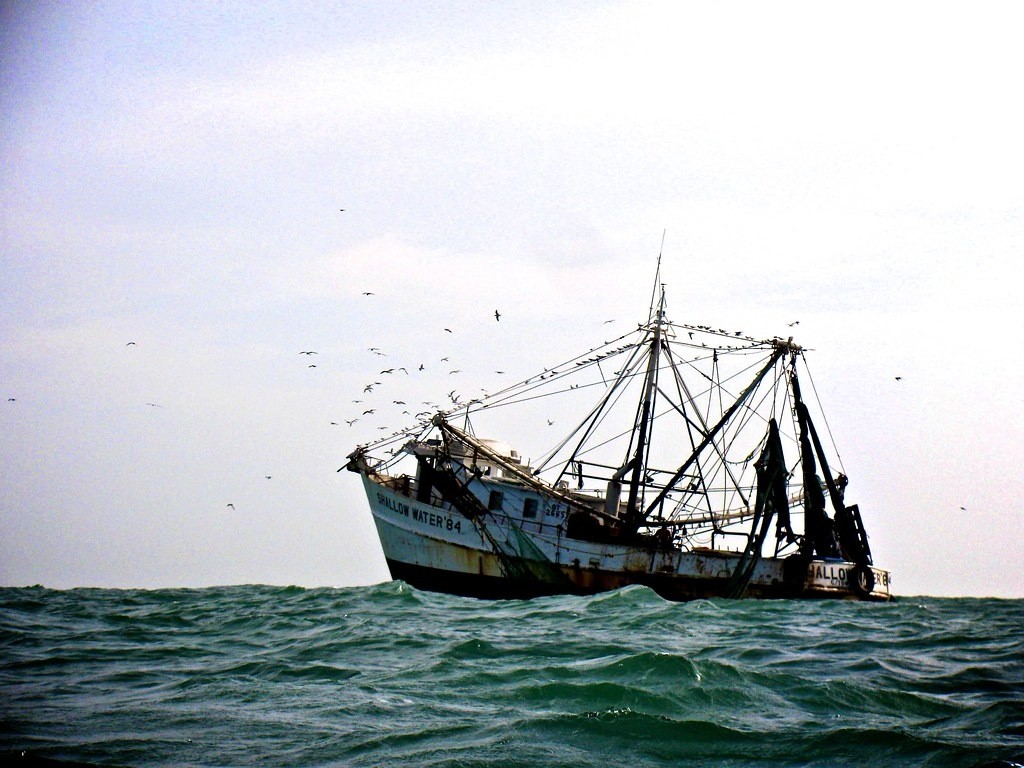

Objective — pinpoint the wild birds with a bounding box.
[5,202,966,519]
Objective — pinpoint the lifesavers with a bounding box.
[850,561,878,597]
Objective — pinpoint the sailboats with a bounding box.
[338,228,893,605]
